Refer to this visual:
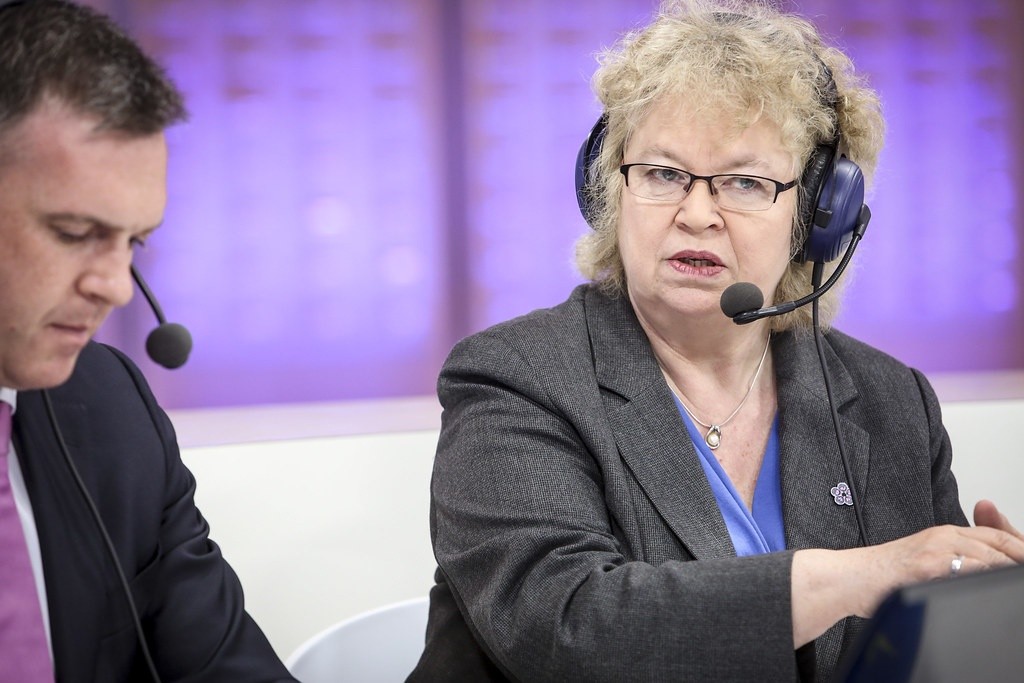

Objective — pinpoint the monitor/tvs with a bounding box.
[823,564,1024,683]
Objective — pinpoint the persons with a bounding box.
[405,0,1024,683]
[0,0,301,683]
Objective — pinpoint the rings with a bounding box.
[950,556,966,578]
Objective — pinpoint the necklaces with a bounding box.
[668,329,771,450]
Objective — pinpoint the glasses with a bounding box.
[618,163,800,213]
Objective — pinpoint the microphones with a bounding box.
[719,235,859,324]
[129,263,191,369]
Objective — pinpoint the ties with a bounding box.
[0,401,53,683]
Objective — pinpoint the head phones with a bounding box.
[576,11,872,263]
